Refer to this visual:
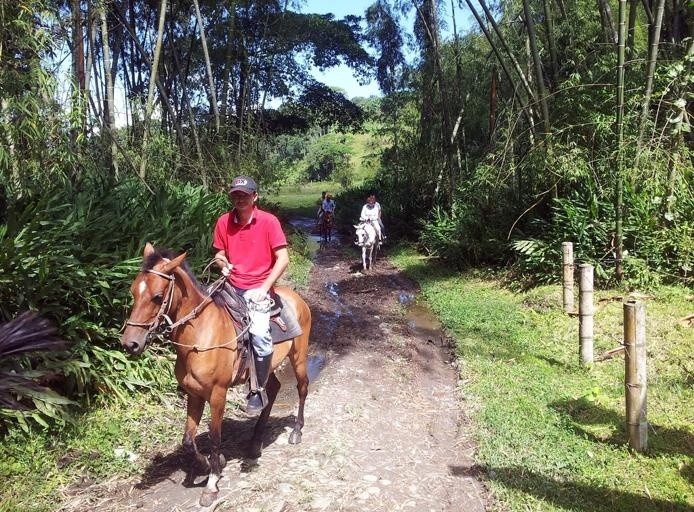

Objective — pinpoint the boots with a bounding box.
[247,352,273,410]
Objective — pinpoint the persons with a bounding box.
[316,192,336,225]
[210,173,292,416]
[316,191,326,219]
[369,194,387,239]
[358,196,383,245]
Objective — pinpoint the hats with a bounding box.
[229,176,256,194]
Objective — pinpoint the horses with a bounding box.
[120,241,312,507]
[319,207,333,244]
[352,222,379,271]
